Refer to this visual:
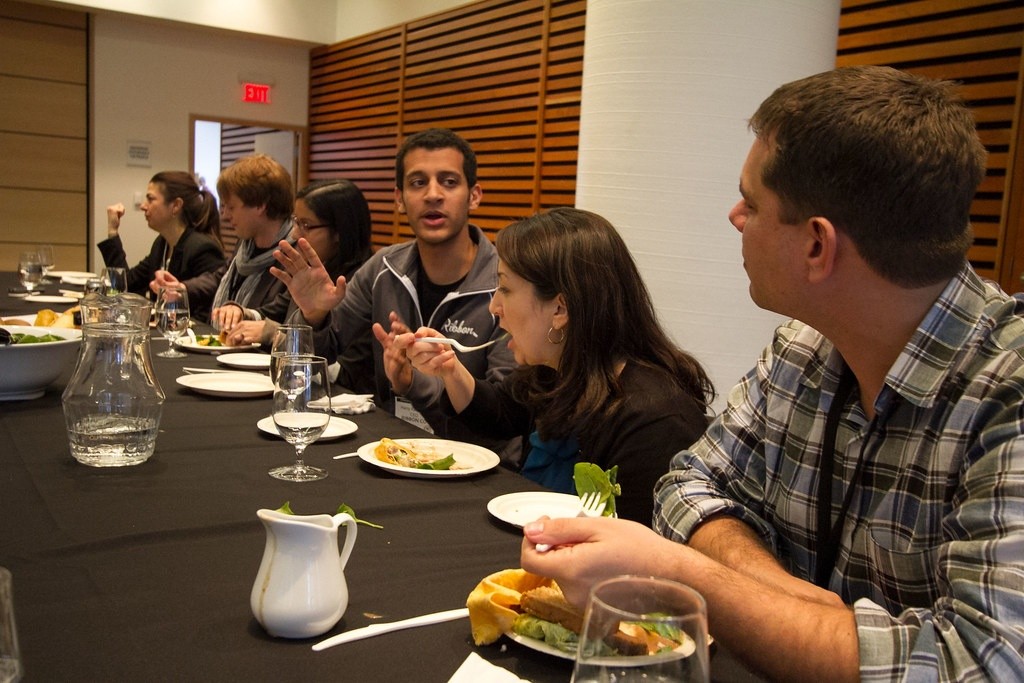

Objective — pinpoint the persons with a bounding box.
[151,153,296,326]
[269,126,525,469]
[96,170,226,325]
[211,178,371,383]
[519,64,1024,683]
[395,208,714,531]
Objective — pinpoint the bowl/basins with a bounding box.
[0,325,83,401]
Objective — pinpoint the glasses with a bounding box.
[290,215,327,235]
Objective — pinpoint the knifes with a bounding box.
[313,599,468,651]
[333,451,358,459]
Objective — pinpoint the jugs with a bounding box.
[61,292,166,466]
[250,509,357,638]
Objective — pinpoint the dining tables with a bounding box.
[0,271,765,683]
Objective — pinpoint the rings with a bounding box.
[241,333,244,338]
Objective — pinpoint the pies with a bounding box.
[33,305,84,330]
[374,437,417,466]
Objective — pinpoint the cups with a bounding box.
[569,575,711,683]
[100,267,127,298]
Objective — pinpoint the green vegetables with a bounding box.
[0,328,66,345]
[415,455,457,471]
[275,500,385,530]
[196,333,222,347]
[511,461,681,659]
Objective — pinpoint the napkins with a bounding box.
[306,392,376,415]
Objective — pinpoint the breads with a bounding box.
[518,584,651,657]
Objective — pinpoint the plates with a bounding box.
[216,352,271,370]
[21,270,97,303]
[357,438,500,478]
[256,412,358,440]
[505,628,696,667]
[486,492,580,528]
[175,373,274,397]
[174,334,261,353]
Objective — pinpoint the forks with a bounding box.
[535,492,606,553]
[394,334,495,352]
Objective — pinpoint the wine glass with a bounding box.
[270,324,314,412]
[17,244,55,300]
[156,286,190,358]
[268,355,331,482]
